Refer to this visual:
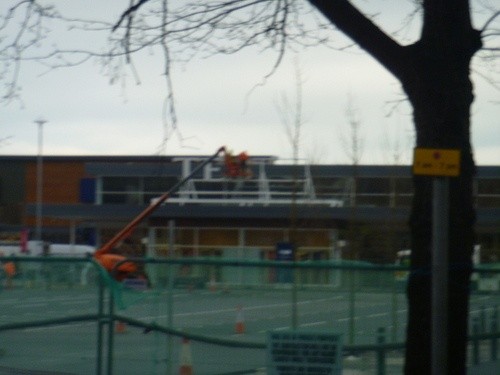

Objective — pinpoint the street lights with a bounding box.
[34,118,47,240]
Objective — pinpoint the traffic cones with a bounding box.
[234,304,247,335]
[114,312,127,333]
[178,336,193,375]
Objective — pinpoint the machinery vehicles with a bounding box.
[84,145,250,289]
